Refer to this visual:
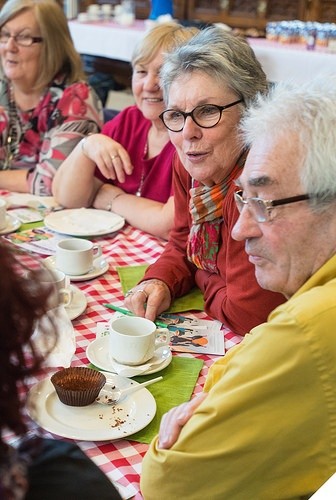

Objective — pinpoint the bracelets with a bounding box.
[107,193,126,212]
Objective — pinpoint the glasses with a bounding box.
[0,32,44,47]
[158,98,246,133]
[233,190,330,223]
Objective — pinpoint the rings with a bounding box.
[111,154,119,159]
[137,289,148,297]
[126,290,133,297]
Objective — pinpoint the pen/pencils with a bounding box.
[102,303,169,328]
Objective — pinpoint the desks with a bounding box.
[67,16,336,94]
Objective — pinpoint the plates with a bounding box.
[0,215,21,235]
[45,255,109,282]
[85,334,173,376]
[26,371,157,442]
[58,284,87,320]
[43,209,125,237]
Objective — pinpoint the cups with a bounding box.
[0,199,8,230]
[109,316,157,365]
[55,238,102,276]
[26,269,73,309]
[78,4,133,27]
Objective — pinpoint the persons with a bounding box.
[0,238,124,500]
[51,25,200,241]
[155,314,194,346]
[125,28,287,336]
[0,0,104,198]
[138,77,336,500]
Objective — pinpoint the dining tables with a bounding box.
[0,189,250,500]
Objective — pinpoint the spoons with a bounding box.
[94,376,163,404]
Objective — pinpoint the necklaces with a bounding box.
[136,141,147,197]
